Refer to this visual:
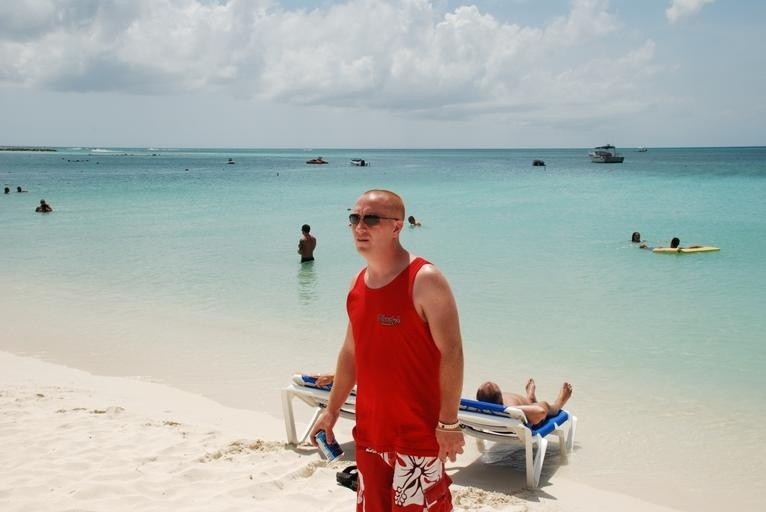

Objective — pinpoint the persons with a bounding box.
[671,238,680,248]
[298,224,316,263]
[310,189,465,512]
[632,232,648,248]
[4,188,9,193]
[17,186,21,192]
[36,199,53,212]
[476,378,573,425]
[408,216,421,225]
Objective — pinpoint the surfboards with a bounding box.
[653,246,719,252]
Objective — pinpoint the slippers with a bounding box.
[336,463,358,492]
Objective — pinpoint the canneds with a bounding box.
[314,430,344,463]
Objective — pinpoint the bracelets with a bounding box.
[435,420,463,433]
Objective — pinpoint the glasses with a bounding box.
[349,213,400,226]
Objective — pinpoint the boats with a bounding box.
[348,159,371,166]
[650,243,721,255]
[588,146,625,163]
[306,159,328,164]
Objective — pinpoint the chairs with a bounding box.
[280,373,576,492]
